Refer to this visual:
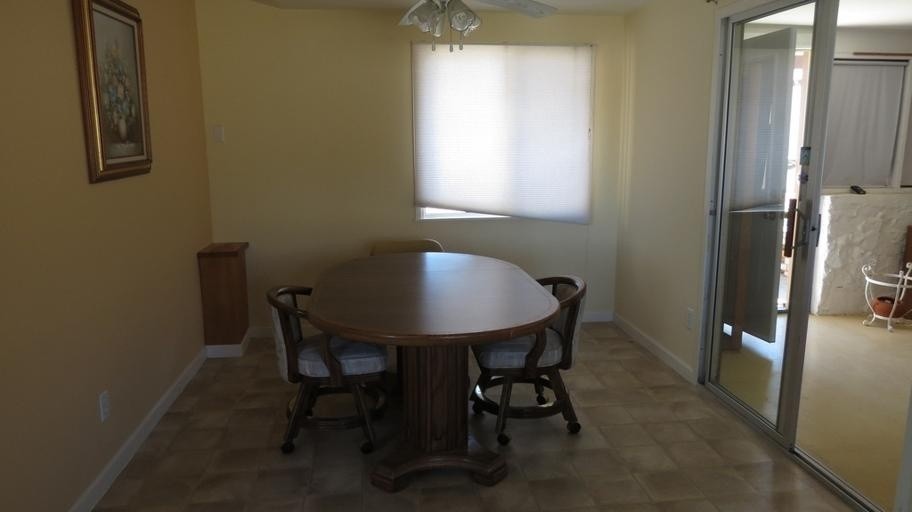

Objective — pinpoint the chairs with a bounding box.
[472,274,585,443]
[267,283,392,456]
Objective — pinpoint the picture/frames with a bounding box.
[71,1,155,185]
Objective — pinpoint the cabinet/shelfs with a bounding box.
[195,242,249,344]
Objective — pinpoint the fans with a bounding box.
[391,0,557,27]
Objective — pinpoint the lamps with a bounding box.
[407,2,483,52]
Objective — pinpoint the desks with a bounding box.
[308,252,561,492]
